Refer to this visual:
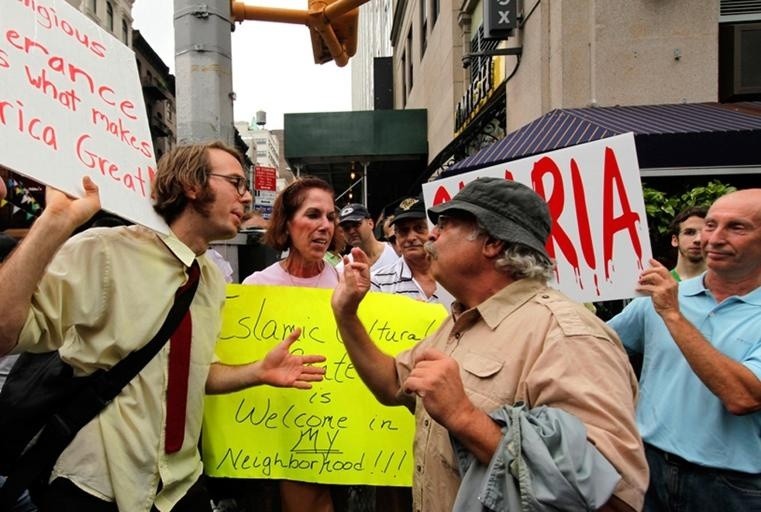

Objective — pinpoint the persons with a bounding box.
[0,140,761,512]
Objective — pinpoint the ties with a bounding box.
[165,284,192,454]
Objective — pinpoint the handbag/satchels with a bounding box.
[0,350,113,511]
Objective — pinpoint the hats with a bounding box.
[388,198,426,227]
[337,204,369,226]
[428,177,553,265]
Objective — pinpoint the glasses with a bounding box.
[206,173,250,196]
[437,213,452,228]
